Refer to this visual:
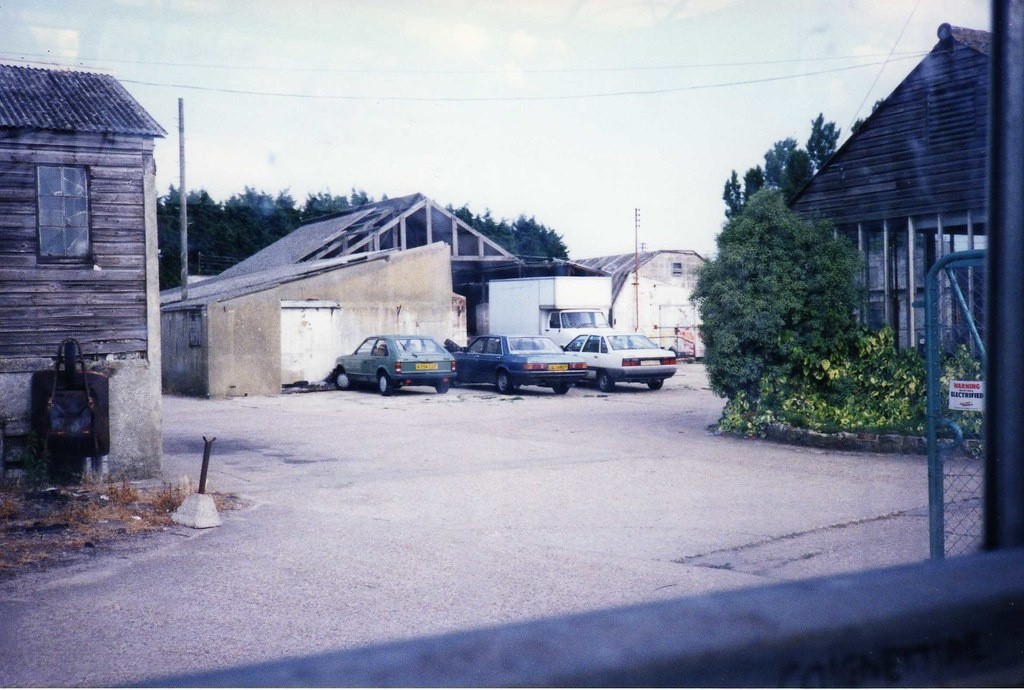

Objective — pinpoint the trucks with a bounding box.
[487,276,617,347]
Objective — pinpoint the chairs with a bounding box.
[487,340,498,354]
[403,344,418,353]
[587,339,599,352]
[376,345,389,356]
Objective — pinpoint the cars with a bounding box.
[562,332,678,393]
[335,334,457,396]
[450,334,590,395]
